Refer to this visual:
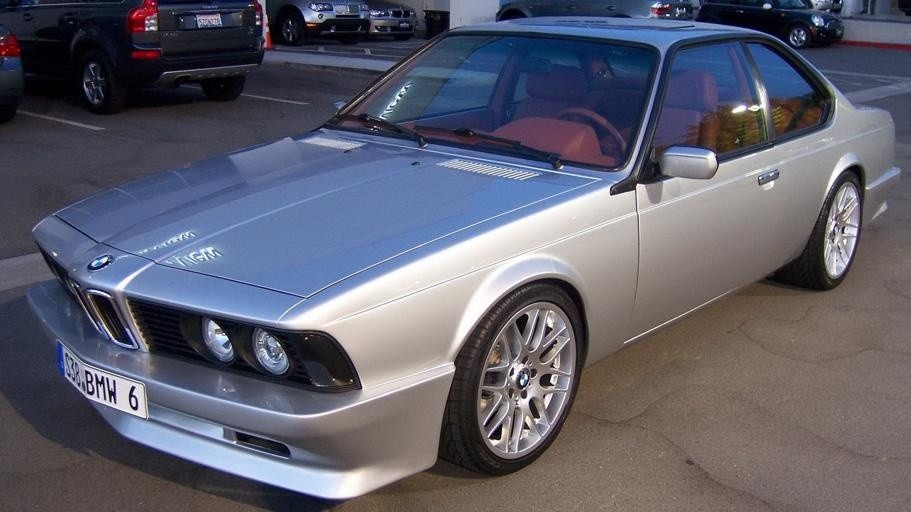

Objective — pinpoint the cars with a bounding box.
[274,0,417,47]
[650,0,844,50]
[0,0,266,123]
[495,0,660,58]
[26,16,902,501]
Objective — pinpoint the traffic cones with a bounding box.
[266,15,275,50]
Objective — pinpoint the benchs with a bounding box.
[582,77,822,155]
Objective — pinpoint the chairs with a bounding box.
[618,68,718,163]
[511,71,585,128]
